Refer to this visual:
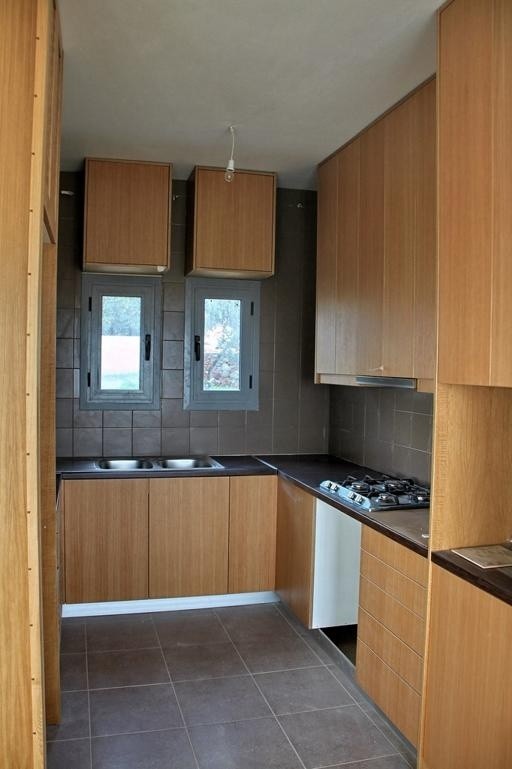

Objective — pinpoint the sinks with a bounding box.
[89,454,154,470]
[155,452,226,471]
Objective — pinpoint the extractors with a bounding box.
[354,374,418,390]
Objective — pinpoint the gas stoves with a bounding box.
[318,472,430,513]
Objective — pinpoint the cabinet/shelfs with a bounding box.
[314,73,434,392]
[184,164,277,281]
[435,2,510,388]
[61,475,276,606]
[82,152,174,277]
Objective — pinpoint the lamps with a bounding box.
[209,119,251,185]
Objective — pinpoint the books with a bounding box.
[450,545,512,569]
